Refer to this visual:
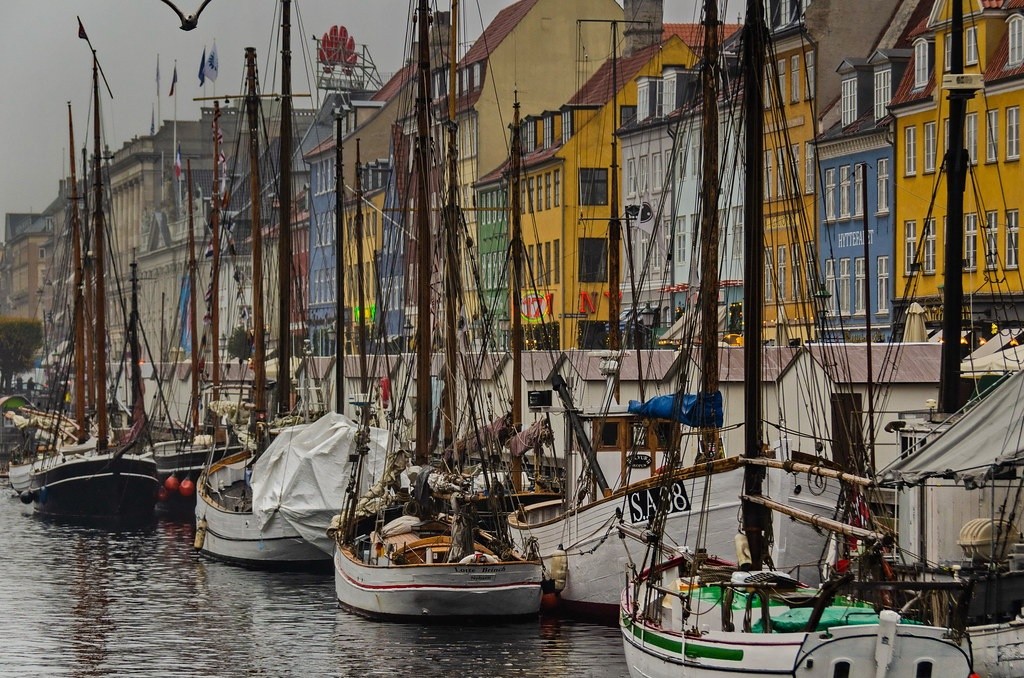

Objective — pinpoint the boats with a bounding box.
[0,0,1024,677]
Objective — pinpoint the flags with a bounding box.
[201,38,219,83]
[191,110,257,374]
[196,44,206,87]
[174,141,183,179]
[147,51,180,137]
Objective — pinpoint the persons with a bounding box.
[15,374,72,413]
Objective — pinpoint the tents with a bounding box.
[959,343,1024,379]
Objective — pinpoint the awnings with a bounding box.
[657,305,729,344]
[40,341,75,365]
[604,303,654,331]
[926,327,1024,362]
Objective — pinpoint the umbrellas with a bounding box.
[170,345,185,361]
[903,302,929,341]
